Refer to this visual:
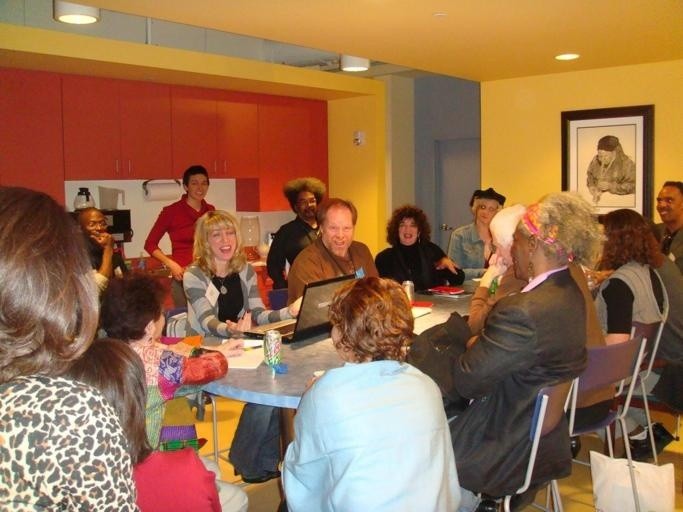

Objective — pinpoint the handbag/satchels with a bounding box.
[591,446,675,512]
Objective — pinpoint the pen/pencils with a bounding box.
[244,345,262,350]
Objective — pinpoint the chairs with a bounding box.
[604,320,665,466]
[484,377,580,512]
[166,305,289,490]
[570,334,647,512]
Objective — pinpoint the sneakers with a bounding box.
[622,432,662,462]
[648,423,674,449]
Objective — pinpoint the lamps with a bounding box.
[54,1,100,25]
[340,55,370,72]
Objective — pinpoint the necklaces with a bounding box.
[211,269,232,296]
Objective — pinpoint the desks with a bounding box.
[202,269,484,501]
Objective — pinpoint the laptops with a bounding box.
[243,275,358,343]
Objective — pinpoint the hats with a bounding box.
[470,188,506,207]
[598,136,619,151]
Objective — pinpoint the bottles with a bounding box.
[263,329,281,366]
[402,281,413,306]
[489,277,498,294]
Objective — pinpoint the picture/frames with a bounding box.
[560,104,654,225]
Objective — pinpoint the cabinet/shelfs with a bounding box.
[0,67,330,211]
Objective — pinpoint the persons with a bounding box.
[99,271,250,512]
[652,181,683,274]
[288,198,380,305]
[266,177,326,290]
[182,211,303,484]
[143,166,216,307]
[0,185,141,512]
[375,206,465,293]
[468,203,615,459]
[594,215,683,464]
[446,192,608,512]
[281,276,482,512]
[79,208,129,295]
[593,209,669,391]
[447,188,506,280]
[586,136,635,207]
[61,339,222,511]
